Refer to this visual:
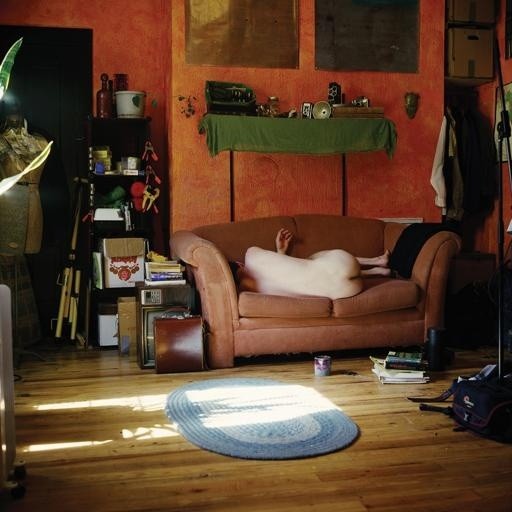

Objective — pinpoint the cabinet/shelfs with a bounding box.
[84,115,152,350]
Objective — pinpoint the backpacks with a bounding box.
[407,364,511,443]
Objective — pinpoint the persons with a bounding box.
[2,131,52,254]
[228,228,391,301]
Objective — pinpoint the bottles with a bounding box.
[426,328,439,365]
[268,97,280,117]
[95,72,115,122]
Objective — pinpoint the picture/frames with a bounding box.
[492,82,512,163]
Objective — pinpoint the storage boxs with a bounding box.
[96,237,150,289]
[445,0,495,25]
[448,27,493,78]
[96,302,119,346]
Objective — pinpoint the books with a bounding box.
[92,251,104,290]
[143,260,188,286]
[367,350,432,386]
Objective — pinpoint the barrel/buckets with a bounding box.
[315,356,331,376]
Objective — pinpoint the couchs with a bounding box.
[167,214,461,369]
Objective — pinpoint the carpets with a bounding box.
[164,377,359,459]
